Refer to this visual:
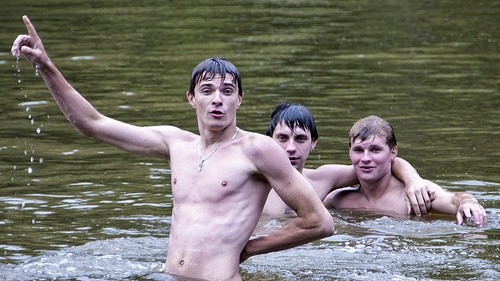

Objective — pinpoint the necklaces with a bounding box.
[197,127,240,173]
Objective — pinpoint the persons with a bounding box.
[322,115,489,229]
[261,102,436,217]
[10,15,335,281]
